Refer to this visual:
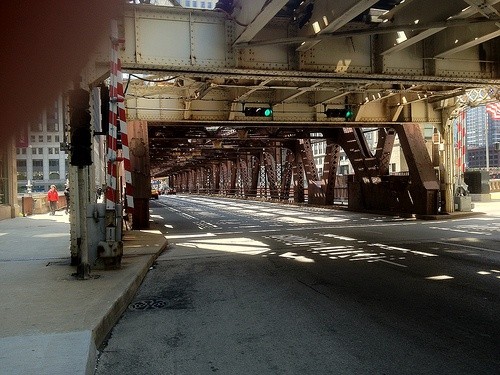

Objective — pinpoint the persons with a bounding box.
[63,181,71,215]
[48,184,58,216]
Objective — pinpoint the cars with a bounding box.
[151,184,177,199]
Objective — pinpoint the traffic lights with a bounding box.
[245,106,273,118]
[326,108,353,119]
